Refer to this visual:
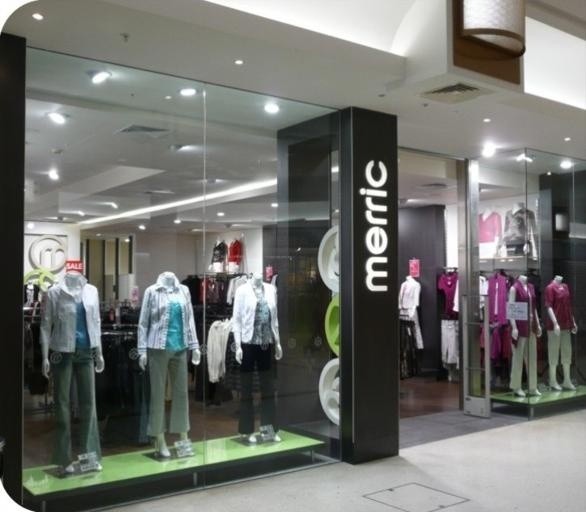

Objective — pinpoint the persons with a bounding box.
[495,200,537,257]
[39,271,105,472]
[545,275,577,391]
[206,239,226,272]
[233,273,282,442]
[24,280,39,306]
[508,275,542,396]
[398,275,424,376]
[477,206,503,258]
[226,238,242,273]
[137,271,200,458]
